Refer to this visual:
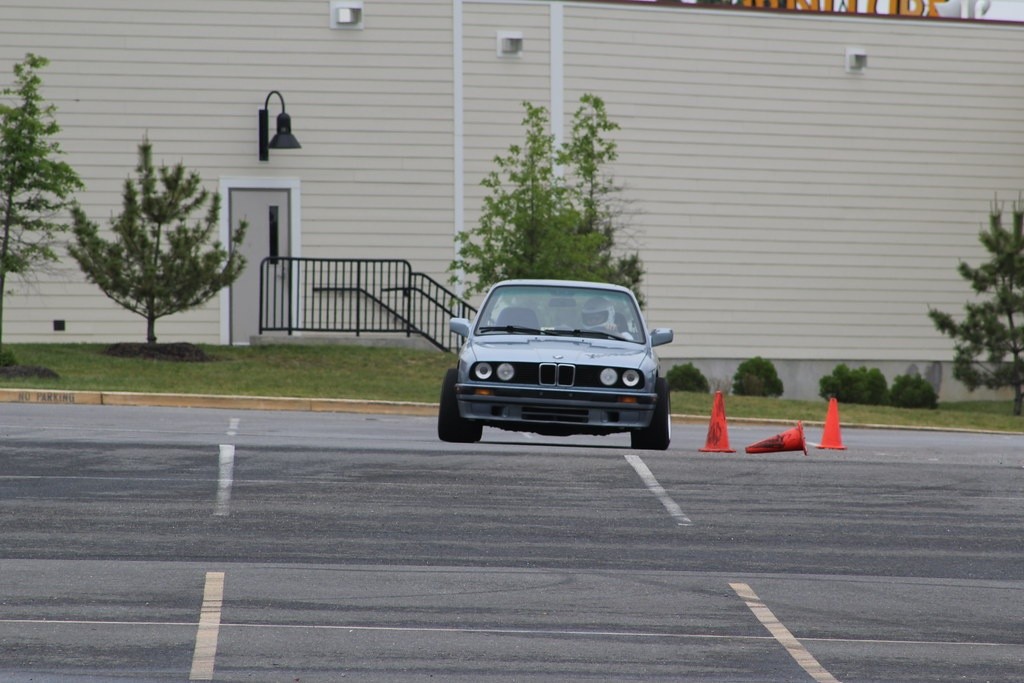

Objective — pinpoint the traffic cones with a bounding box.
[697,390,737,453]
[811,395,848,450]
[744,418,808,456]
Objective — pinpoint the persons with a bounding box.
[582,298,634,341]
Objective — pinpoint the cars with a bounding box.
[437,278,675,450]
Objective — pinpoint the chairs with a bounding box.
[497,307,538,330]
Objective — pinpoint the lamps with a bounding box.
[259,90,301,161]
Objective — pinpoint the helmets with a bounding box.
[580,296,616,332]
